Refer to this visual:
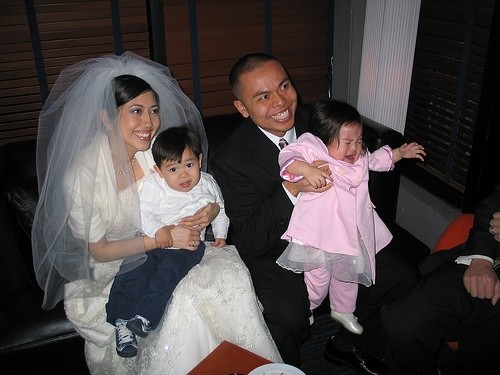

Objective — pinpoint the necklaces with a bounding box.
[114,155,138,175]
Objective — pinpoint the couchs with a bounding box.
[0,106,430,375]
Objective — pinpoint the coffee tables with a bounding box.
[185,341,273,375]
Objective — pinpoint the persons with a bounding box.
[209,52,500,375]
[32,50,282,375]
[104,127,230,357]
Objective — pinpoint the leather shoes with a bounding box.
[324,335,385,375]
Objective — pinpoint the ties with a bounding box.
[278,138,289,150]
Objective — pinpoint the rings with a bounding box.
[491,226,495,234]
[190,241,196,247]
[198,224,202,230]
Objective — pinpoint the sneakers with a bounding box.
[114,319,137,358]
[126,315,153,338]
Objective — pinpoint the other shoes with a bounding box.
[330,311,363,334]
[309,310,314,325]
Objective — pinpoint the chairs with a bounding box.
[434,213,473,351]
